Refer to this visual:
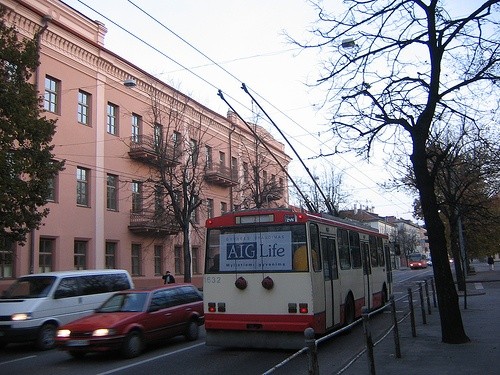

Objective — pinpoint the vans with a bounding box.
[0,267,137,351]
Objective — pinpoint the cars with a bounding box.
[47,281,205,363]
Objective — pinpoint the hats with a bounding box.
[166,271,171,273]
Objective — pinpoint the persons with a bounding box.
[348,242,361,267]
[162,271,176,284]
[488,255,495,271]
[209,253,219,272]
[293,237,318,271]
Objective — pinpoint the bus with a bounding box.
[199,82,395,352]
[406,253,433,270]
